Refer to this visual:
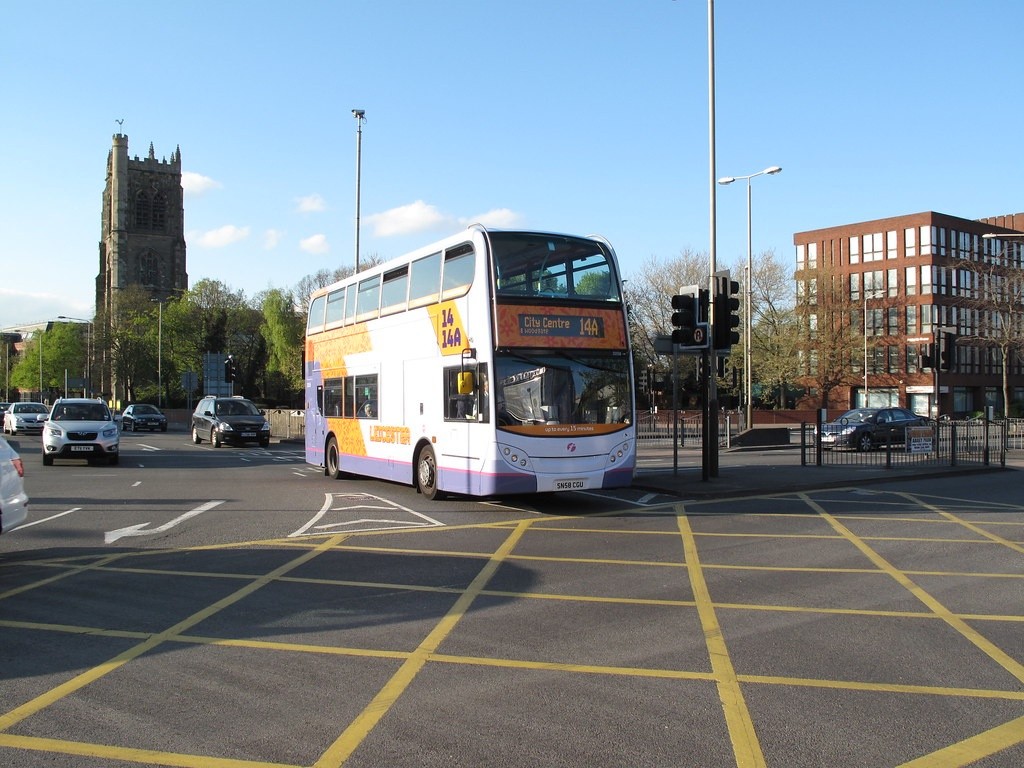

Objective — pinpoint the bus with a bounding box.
[305,223,636,502]
[500,366,575,426]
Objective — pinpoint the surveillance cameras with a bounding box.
[351,110,365,118]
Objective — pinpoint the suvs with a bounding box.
[37,397,122,466]
[191,396,271,447]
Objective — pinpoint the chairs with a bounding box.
[335,399,378,417]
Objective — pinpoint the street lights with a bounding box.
[58,316,91,399]
[865,290,886,409]
[15,329,43,403]
[718,166,783,428]
[151,298,161,406]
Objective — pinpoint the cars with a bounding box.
[3,402,50,436]
[121,405,168,432]
[0,402,13,426]
[0,436,28,535]
[812,408,928,452]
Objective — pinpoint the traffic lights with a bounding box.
[231,362,237,381]
[715,269,740,349]
[671,285,709,349]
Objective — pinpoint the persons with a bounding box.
[56,406,102,419]
[553,382,589,424]
[216,403,239,414]
[365,404,376,417]
[457,393,472,418]
